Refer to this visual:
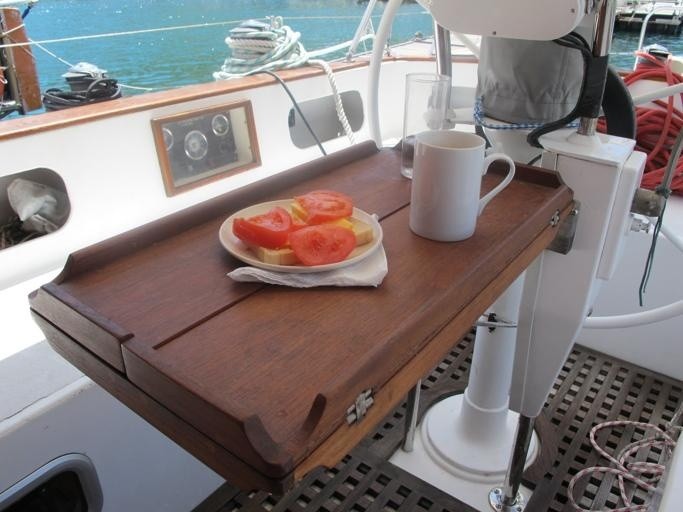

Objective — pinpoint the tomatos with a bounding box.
[291,223,358,267]
[230,207,294,247]
[295,190,354,222]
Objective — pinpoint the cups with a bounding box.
[409,129,518,244]
[401,70,451,180]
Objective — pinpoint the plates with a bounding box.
[217,192,383,273]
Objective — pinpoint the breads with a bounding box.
[242,211,373,266]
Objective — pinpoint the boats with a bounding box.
[0,0,683,512]
[615,0,683,36]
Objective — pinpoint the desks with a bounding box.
[24,134,579,497]
